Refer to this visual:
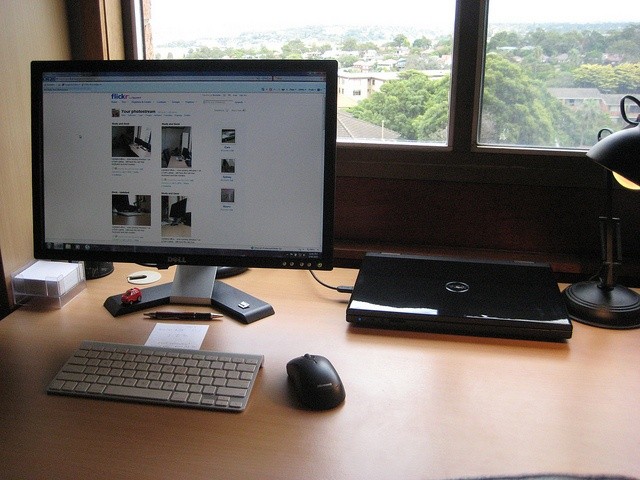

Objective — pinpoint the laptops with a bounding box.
[346,257,572,341]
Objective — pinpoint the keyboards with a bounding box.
[45,341,265,413]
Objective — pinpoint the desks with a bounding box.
[1,260,640,478]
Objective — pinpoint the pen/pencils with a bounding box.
[143,312,224,320]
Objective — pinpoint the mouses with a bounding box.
[287,354,346,410]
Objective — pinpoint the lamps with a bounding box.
[561,94,640,328]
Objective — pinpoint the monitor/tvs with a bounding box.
[29,57,339,326]
